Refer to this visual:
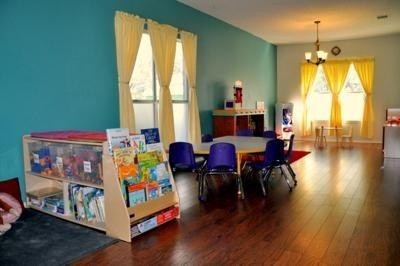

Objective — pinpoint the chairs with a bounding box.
[236,130,254,137]
[201,134,214,143]
[267,134,298,186]
[314,128,327,147]
[341,126,354,150]
[244,139,293,196]
[201,142,245,201]
[168,141,205,201]
[261,130,278,139]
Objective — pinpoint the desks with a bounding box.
[187,135,288,196]
[317,127,342,148]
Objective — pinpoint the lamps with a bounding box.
[304,19,328,66]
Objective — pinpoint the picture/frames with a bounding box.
[223,97,235,111]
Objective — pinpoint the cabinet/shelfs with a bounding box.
[23,131,107,234]
[275,102,294,140]
[211,109,265,137]
[103,141,181,242]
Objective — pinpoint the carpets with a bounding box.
[240,149,312,166]
[0,208,120,266]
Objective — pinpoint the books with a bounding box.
[26,189,64,215]
[64,181,105,225]
[106,126,172,206]
[130,206,179,237]
